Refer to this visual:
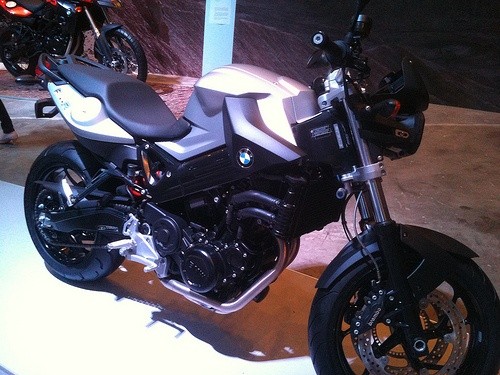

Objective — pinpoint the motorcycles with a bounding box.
[0,1,148,87]
[24,0,499,375]
